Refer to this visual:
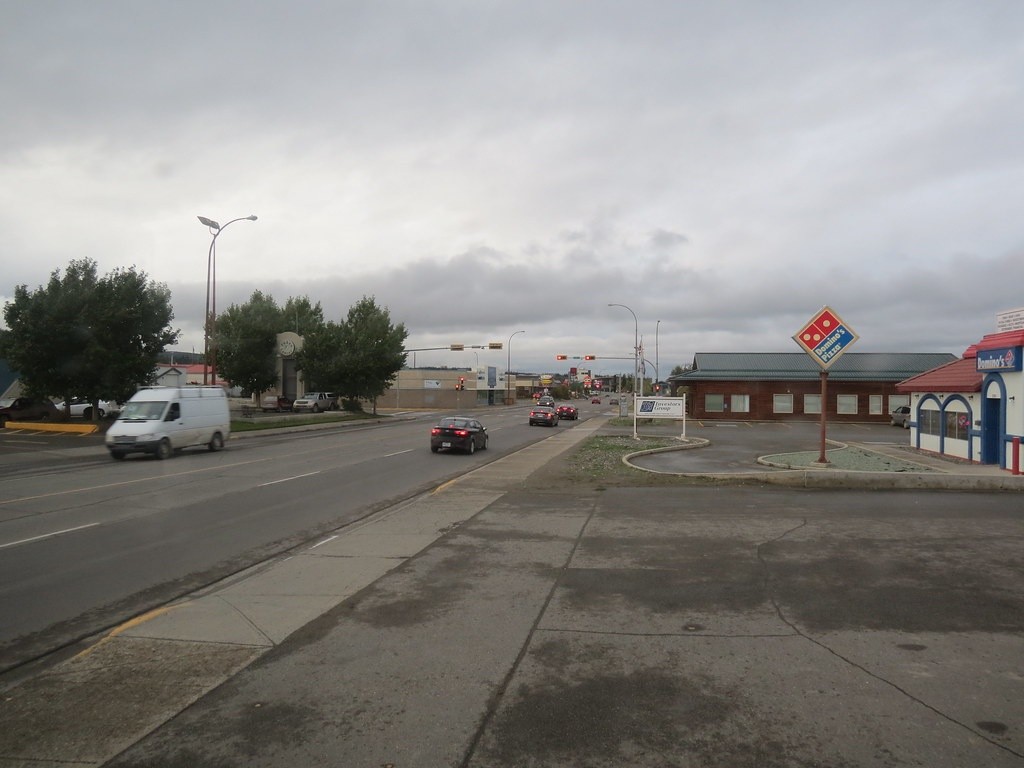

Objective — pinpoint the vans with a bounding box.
[105,385,231,459]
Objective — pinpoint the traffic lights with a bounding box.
[656,385,659,391]
[461,377,464,390]
[455,384,458,390]
[557,355,566,359]
[585,355,595,360]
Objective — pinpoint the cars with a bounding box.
[431,416,487,456]
[557,403,579,420]
[592,397,600,403]
[262,395,293,412]
[610,397,619,405]
[891,406,911,428]
[529,406,560,427]
[538,396,555,408]
[0,395,56,428]
[56,396,110,419]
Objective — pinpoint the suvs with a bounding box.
[294,392,338,412]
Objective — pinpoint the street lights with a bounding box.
[508,330,525,405]
[197,215,257,386]
[608,303,637,393]
